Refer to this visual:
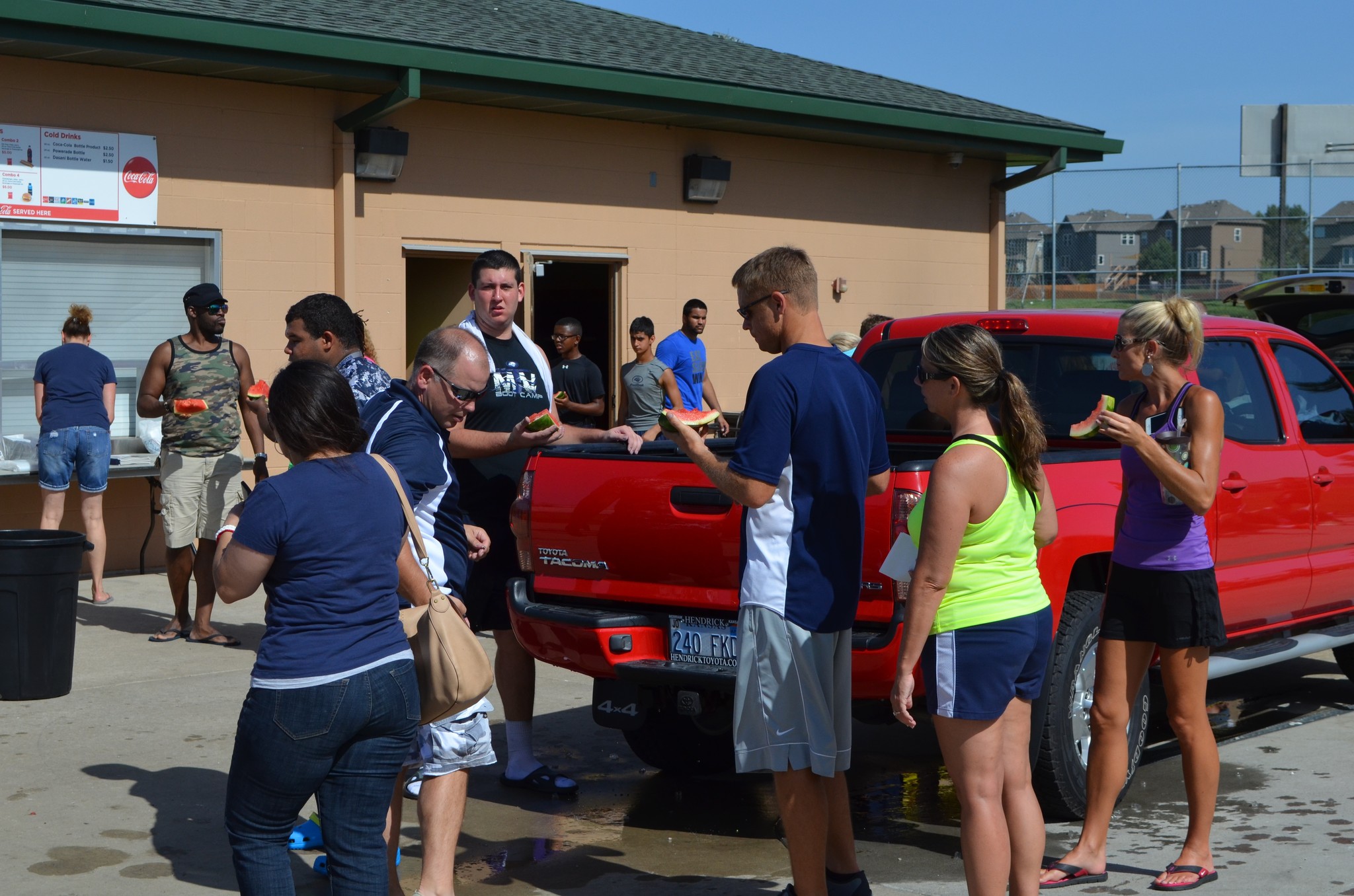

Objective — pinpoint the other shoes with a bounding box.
[776,866,873,896]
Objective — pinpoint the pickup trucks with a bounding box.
[500,305,1353,827]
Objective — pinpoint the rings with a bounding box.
[893,712,900,716]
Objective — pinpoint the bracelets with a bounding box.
[215,526,236,545]
[253,451,269,461]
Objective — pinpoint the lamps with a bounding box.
[681,150,731,207]
[354,124,411,184]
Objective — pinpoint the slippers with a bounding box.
[287,812,324,850]
[149,629,191,642]
[500,764,579,793]
[313,846,401,876]
[185,633,241,645]
[1038,860,1108,888]
[1154,862,1217,891]
[92,592,114,604]
[403,768,425,801]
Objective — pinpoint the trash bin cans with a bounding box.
[0,529,95,701]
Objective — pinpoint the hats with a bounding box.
[183,283,228,314]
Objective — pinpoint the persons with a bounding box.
[33,302,119,604]
[212,359,421,896]
[361,321,493,896]
[547,313,608,428]
[658,243,890,896]
[402,248,644,800]
[1040,295,1225,891]
[890,323,1059,896]
[135,282,270,647]
[611,298,731,443]
[284,292,395,410]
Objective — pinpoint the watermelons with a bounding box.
[247,379,270,401]
[553,391,565,399]
[658,407,720,431]
[174,398,209,415]
[525,409,559,432]
[1070,395,1115,439]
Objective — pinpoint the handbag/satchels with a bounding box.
[365,453,494,725]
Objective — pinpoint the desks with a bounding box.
[0,450,256,576]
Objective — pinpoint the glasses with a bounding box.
[736,286,801,320]
[551,334,578,341]
[420,359,490,401]
[196,304,228,315]
[917,366,951,385]
[1113,333,1166,352]
